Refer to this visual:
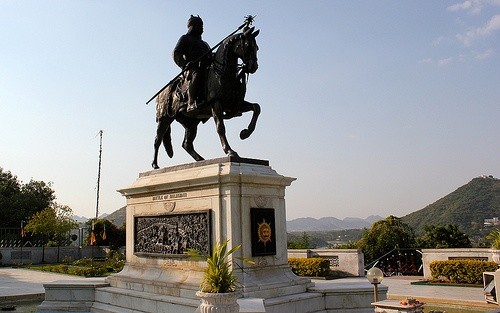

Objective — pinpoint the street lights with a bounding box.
[367,267,384,309]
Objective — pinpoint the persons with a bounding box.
[172,12,222,114]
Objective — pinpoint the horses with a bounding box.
[151,26,261,169]
